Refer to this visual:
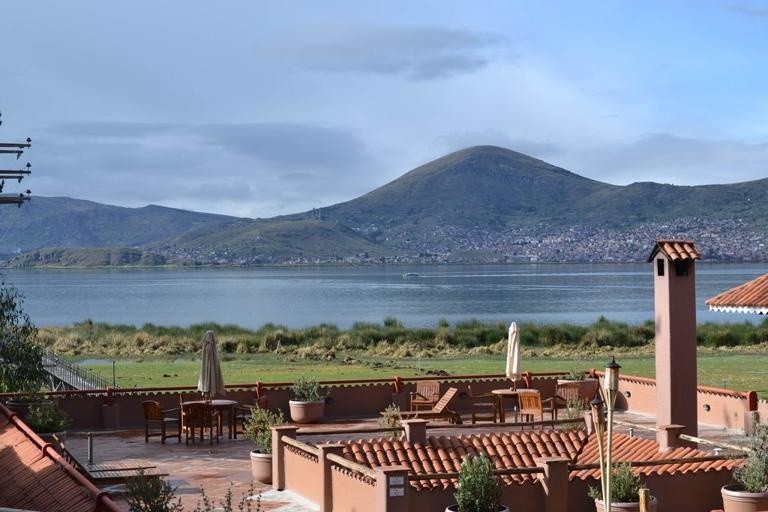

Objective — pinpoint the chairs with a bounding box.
[139,400,270,446]
[52,433,169,494]
[380,377,559,426]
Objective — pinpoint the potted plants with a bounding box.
[585,462,657,512]
[557,367,598,407]
[288,376,326,423]
[443,453,511,512]
[246,403,288,483]
[717,414,768,511]
[0,286,69,453]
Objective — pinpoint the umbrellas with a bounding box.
[197,330,226,434]
[506,320,523,423]
